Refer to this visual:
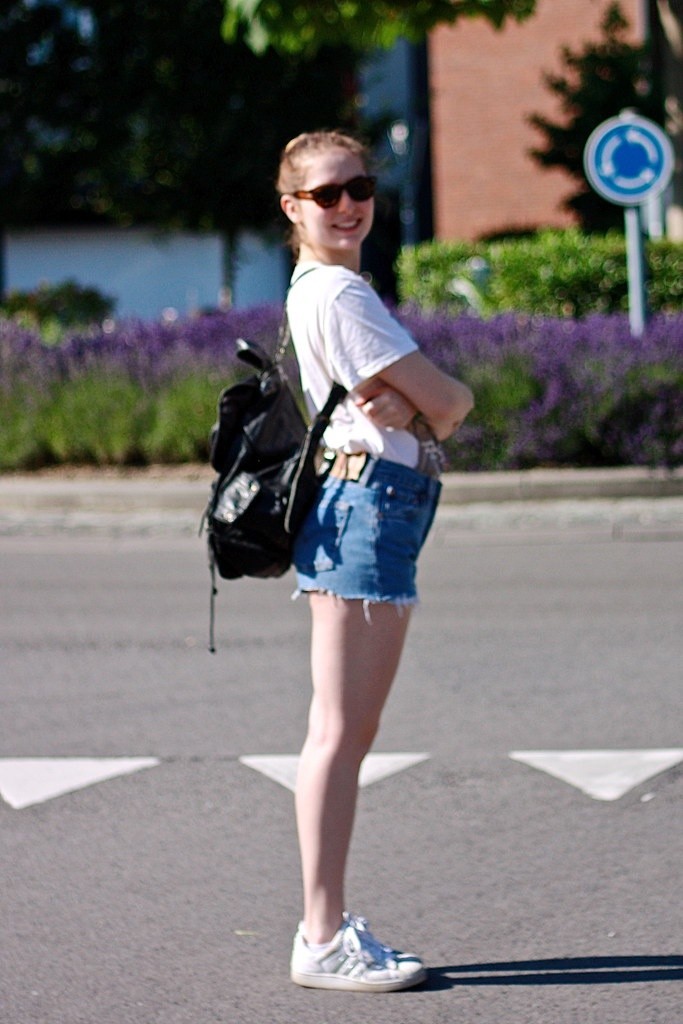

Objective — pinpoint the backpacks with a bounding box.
[198,268,348,654]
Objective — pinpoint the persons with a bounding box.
[278,133,475,993]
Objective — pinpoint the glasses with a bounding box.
[293,176,377,208]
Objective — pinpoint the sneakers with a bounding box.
[290,912,426,992]
